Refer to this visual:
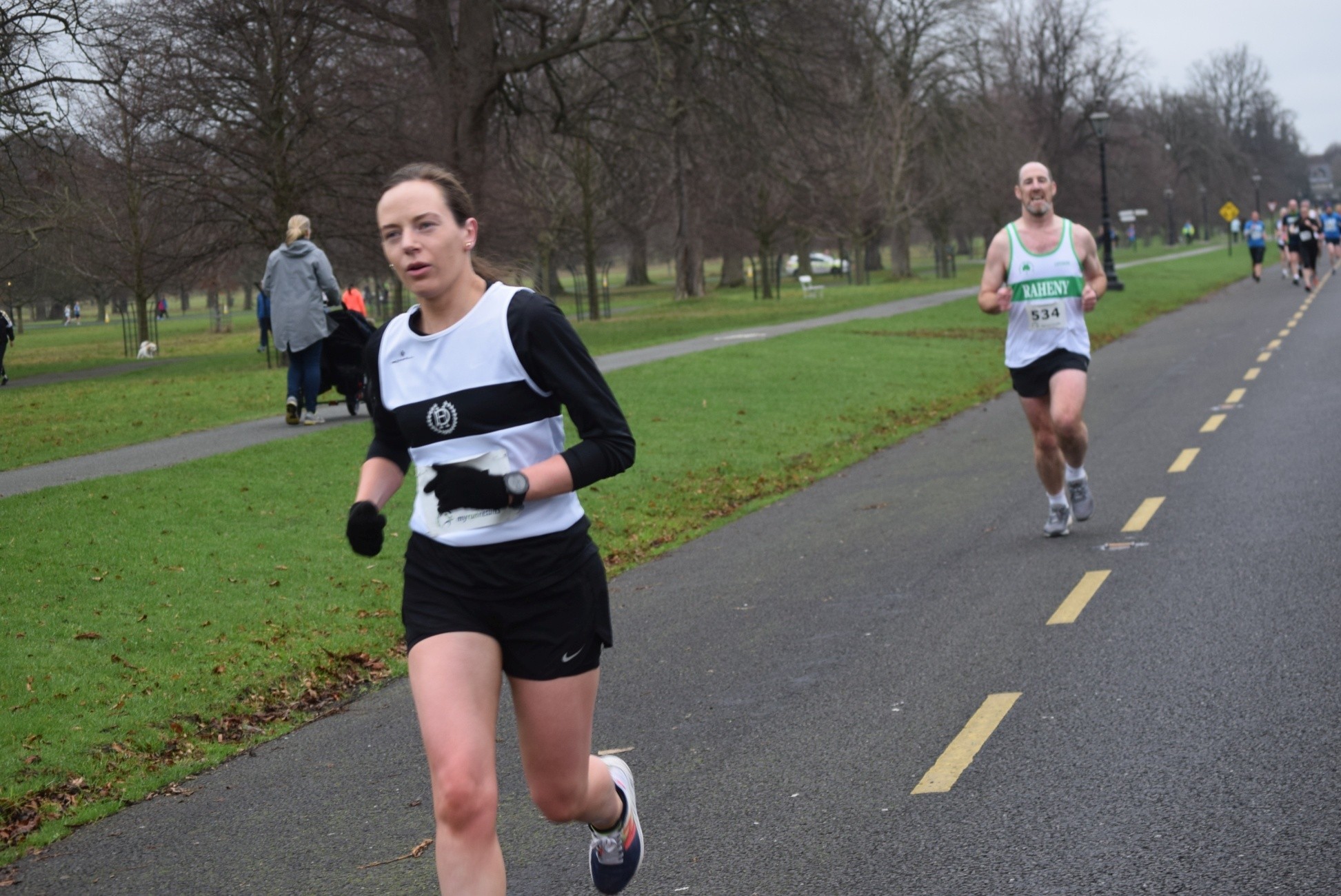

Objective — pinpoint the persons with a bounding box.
[978,159,1109,536]
[0,311,14,387]
[155,299,165,321]
[137,341,157,361]
[160,294,169,319]
[256,278,274,350]
[1096,196,1341,291]
[342,161,651,894]
[63,303,71,327]
[264,214,348,425]
[340,283,367,321]
[73,300,81,326]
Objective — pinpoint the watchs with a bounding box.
[502,470,530,511]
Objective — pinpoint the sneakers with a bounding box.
[1067,469,1094,519]
[304,412,325,425]
[286,398,299,423]
[1045,500,1073,536]
[586,753,645,896]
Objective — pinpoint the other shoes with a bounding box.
[1248,263,1341,293]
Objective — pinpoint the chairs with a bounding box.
[799,275,825,300]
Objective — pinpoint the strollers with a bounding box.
[318,298,379,418]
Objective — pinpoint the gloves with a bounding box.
[424,462,508,513]
[343,501,385,557]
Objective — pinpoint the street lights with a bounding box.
[1200,187,1213,240]
[1250,169,1263,213]
[1164,188,1178,245]
[1089,95,1125,290]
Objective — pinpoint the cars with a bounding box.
[787,254,852,276]
